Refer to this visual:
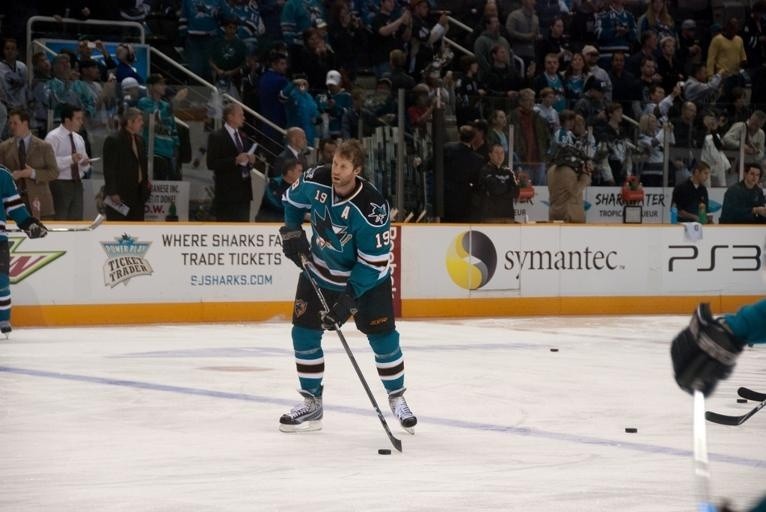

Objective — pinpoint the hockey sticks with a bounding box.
[704,398,766,425]
[0,213,106,233]
[301,255,401,452]
[738,387,766,401]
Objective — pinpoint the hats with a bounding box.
[312,17,328,30]
[325,69,343,87]
[583,44,598,56]
[377,76,394,89]
[120,77,148,91]
[681,19,697,30]
[148,72,168,83]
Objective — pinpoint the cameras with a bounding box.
[347,12,353,18]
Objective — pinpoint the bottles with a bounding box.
[671,202,678,223]
[698,197,707,224]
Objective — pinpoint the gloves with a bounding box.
[279,225,315,271]
[19,217,48,240]
[319,281,361,332]
[669,300,749,399]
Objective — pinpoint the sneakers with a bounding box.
[0,320,13,333]
[280,385,326,425]
[388,385,416,429]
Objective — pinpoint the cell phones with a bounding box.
[87,42,96,48]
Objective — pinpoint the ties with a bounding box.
[17,139,28,190]
[233,130,250,175]
[68,131,81,184]
[131,135,143,183]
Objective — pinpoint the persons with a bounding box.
[0,164,48,333]
[671,296,766,512]
[278,138,416,427]
